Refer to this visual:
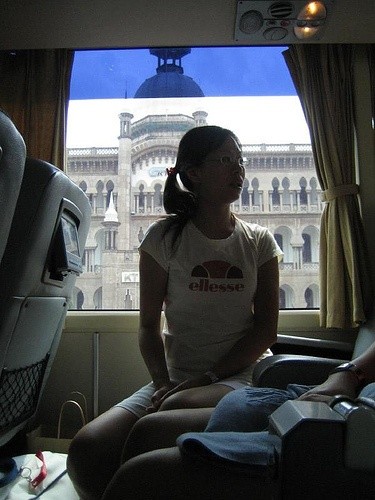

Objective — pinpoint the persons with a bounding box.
[66,126,284,500]
[103,340,375,500]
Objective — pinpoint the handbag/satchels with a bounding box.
[26,390,87,454]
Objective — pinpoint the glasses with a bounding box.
[202,155,244,169]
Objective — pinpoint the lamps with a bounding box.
[234,0,329,41]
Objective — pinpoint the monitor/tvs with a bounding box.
[57,211,83,274]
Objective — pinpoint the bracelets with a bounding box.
[203,371,219,382]
[328,361,365,391]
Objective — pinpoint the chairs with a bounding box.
[1,156,91,447]
[251,319,375,500]
[1,111,27,271]
